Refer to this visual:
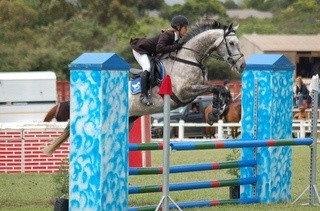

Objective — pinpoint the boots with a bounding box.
[140,70,152,106]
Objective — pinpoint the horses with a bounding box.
[202,99,242,141]
[43,100,70,122]
[293,96,312,121]
[42,18,248,158]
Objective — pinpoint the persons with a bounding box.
[129,15,189,106]
[189,106,199,113]
[218,80,233,120]
[293,77,311,112]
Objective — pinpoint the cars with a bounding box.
[150,95,213,139]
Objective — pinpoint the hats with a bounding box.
[224,79,230,85]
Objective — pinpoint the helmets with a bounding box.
[171,16,189,27]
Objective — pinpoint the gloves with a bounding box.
[175,43,182,50]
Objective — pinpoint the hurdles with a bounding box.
[128,79,258,211]
[159,75,320,211]
